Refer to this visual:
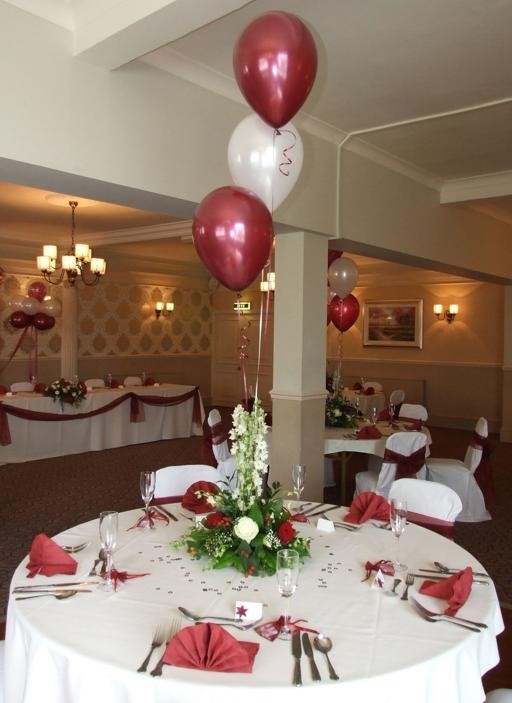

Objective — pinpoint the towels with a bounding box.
[145,377,156,386]
[419,565,473,616]
[343,489,396,524]
[181,479,222,515]
[34,382,46,393]
[159,620,260,674]
[109,378,120,388]
[25,531,80,578]
[0,384,8,394]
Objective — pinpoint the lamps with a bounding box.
[259,271,275,302]
[154,300,175,320]
[432,303,459,324]
[35,199,108,288]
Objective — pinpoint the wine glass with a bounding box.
[275,548,301,637]
[389,498,408,574]
[138,470,158,536]
[95,510,119,594]
[353,397,396,431]
[289,463,308,521]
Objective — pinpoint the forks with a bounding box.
[412,597,489,633]
[137,617,180,678]
[195,617,266,633]
[400,574,414,601]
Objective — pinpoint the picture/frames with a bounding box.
[361,297,423,350]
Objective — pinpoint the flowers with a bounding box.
[171,394,312,580]
[42,374,88,410]
[324,362,361,429]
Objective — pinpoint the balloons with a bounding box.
[190,185,273,292]
[8,282,61,330]
[232,10,318,129]
[226,110,304,214]
[327,248,359,333]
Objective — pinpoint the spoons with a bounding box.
[14,587,79,601]
[435,561,493,578]
[314,632,339,682]
[178,603,245,622]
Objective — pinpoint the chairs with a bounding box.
[351,380,490,543]
[137,462,222,508]
[10,381,36,395]
[207,406,269,497]
[84,377,106,389]
[123,375,143,386]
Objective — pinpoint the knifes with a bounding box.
[409,569,490,585]
[151,504,179,526]
[291,628,321,687]
[301,501,345,519]
[11,581,99,593]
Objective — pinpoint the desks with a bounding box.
[0,382,208,467]
